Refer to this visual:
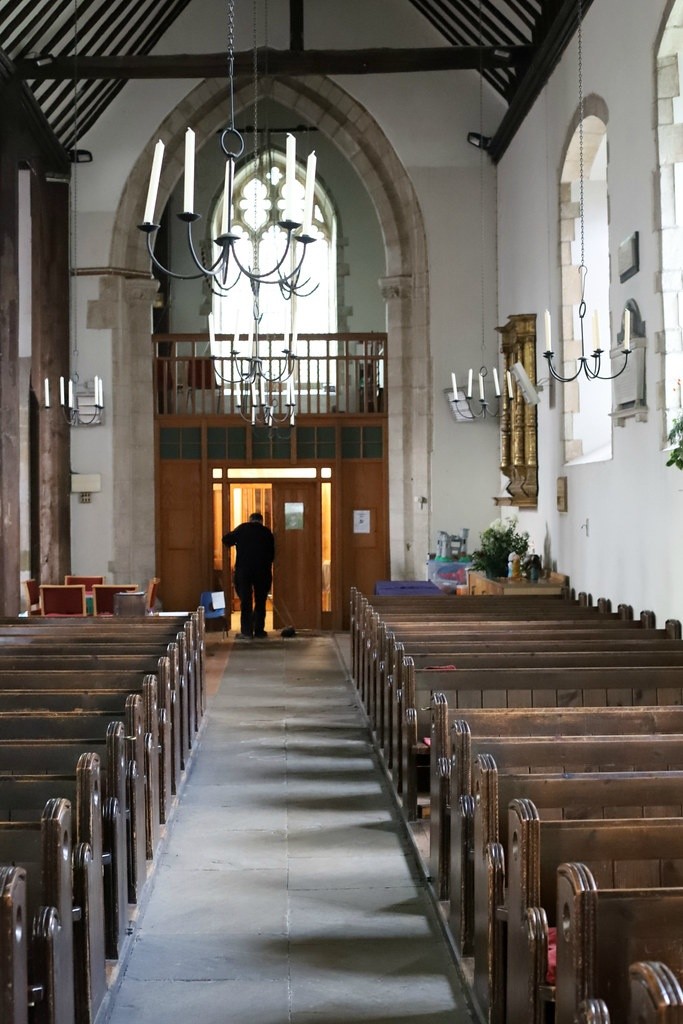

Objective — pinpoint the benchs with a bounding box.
[349,587,682,1024]
[0,605,209,1024]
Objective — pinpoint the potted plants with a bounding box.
[466,514,531,578]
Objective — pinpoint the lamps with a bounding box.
[26,51,55,68]
[67,149,93,163]
[467,132,493,150]
[494,47,524,65]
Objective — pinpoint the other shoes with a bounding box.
[234,632,255,638]
[257,630,267,638]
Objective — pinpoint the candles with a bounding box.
[68,379,73,408]
[283,131,299,221]
[143,138,165,223]
[507,369,513,399]
[300,150,318,237]
[94,375,99,405]
[492,366,502,396]
[451,372,458,400]
[44,378,50,407]
[467,367,473,397]
[478,372,484,399]
[543,305,631,357]
[98,378,104,407]
[60,375,65,405]
[183,127,194,214]
[206,309,301,430]
[219,156,236,233]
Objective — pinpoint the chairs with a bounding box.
[64,575,107,594]
[200,590,229,638]
[145,577,160,608]
[39,584,88,618]
[23,579,41,617]
[91,583,138,616]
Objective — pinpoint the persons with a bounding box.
[220,511,276,638]
